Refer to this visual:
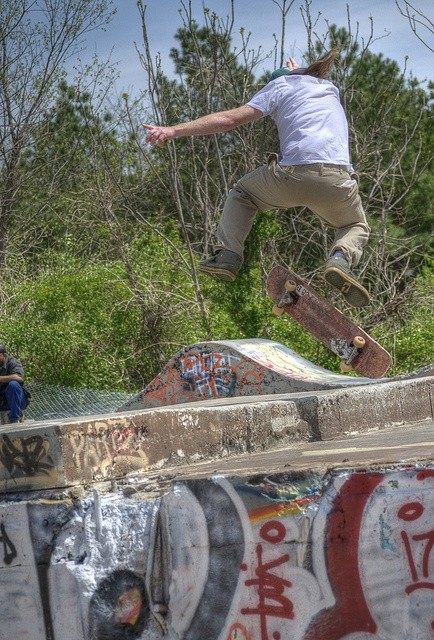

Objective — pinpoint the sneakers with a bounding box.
[324,258,369,307]
[198,249,242,281]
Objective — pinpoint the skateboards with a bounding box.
[266,265,392,380]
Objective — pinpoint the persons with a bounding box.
[142,45,371,308]
[0,345,34,424]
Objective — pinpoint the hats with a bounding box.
[269,67,290,81]
[0,345,5,352]
